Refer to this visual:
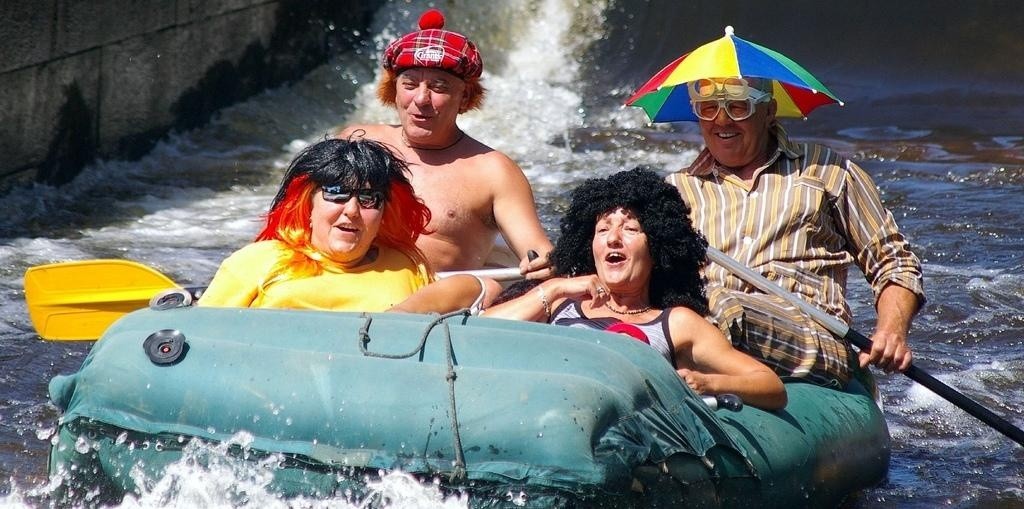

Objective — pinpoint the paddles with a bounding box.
[24,250,541,341]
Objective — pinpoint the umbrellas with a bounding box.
[618,23,846,130]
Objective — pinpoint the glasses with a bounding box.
[689,93,772,121]
[321,184,384,211]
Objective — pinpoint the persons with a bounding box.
[475,163,789,422]
[181,130,506,326]
[329,8,564,292]
[661,75,932,391]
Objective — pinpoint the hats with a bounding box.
[383,29,484,80]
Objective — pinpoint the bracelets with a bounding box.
[534,284,552,319]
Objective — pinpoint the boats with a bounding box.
[45,302,894,507]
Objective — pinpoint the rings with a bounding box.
[595,287,606,294]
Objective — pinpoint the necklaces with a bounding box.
[602,297,655,314]
[396,127,465,151]
